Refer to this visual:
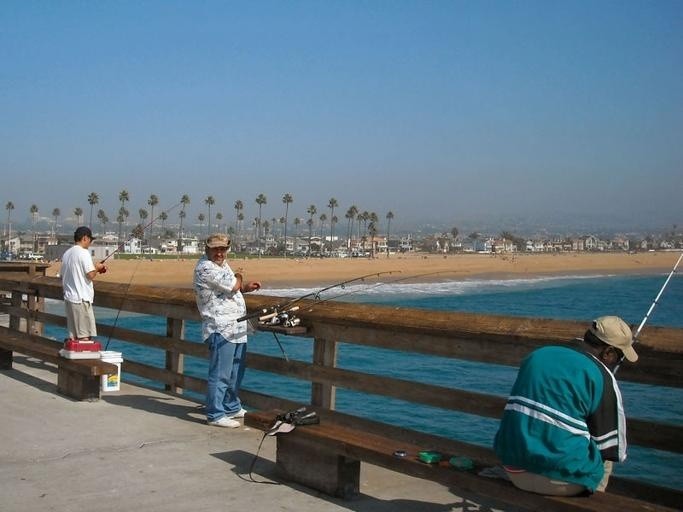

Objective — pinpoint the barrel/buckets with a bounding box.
[98,349,125,391]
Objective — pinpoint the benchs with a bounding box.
[242,405,683,512]
[0,330,117,401]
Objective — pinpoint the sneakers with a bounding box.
[207,415,241,428]
[227,407,248,418]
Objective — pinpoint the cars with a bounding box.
[248,248,264,255]
[0,251,44,260]
[297,249,371,258]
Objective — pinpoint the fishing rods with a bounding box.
[256,253,472,328]
[611,251,683,376]
[99,194,192,275]
[103,248,146,352]
[236,270,404,322]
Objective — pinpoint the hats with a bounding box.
[74,226,96,239]
[205,232,232,249]
[588,316,639,363]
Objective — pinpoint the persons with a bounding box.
[491,316,639,495]
[192,234,261,428]
[60,227,109,343]
